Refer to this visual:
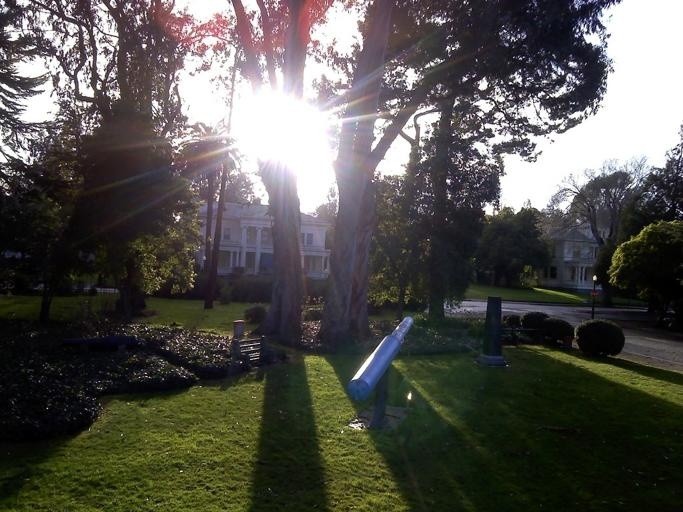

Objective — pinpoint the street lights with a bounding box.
[591,274,598,320]
